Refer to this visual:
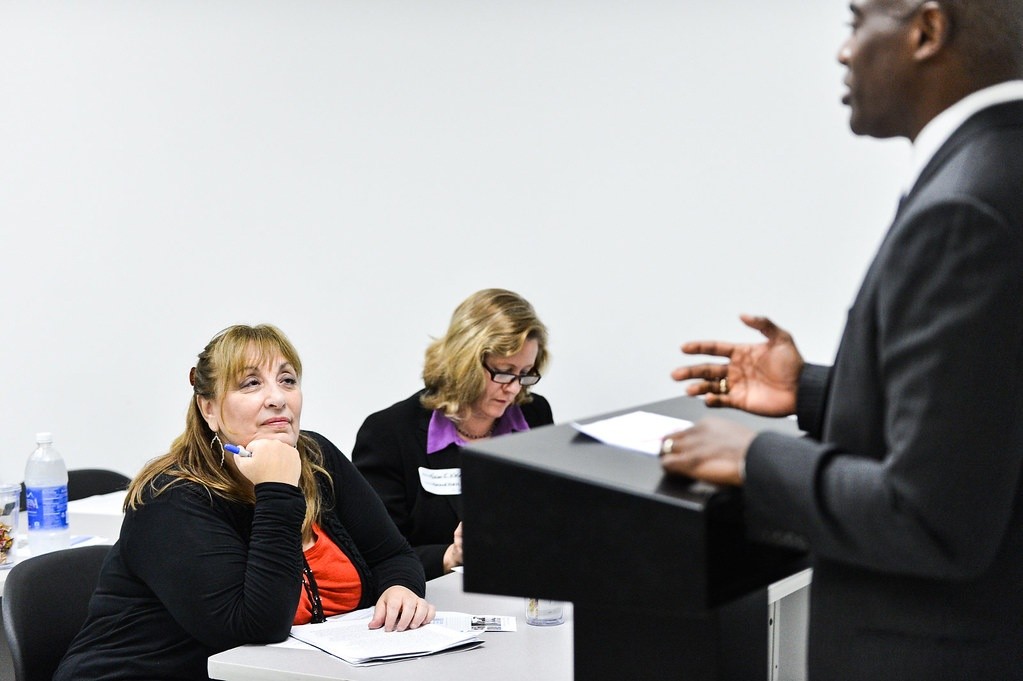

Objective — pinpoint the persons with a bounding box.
[55,321,435,680]
[657,0,1023,681]
[351,288,557,581]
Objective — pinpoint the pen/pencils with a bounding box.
[223,443,252,458]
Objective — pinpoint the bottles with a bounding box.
[25,433,71,553]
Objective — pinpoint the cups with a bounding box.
[525,598,564,626]
[0,483,22,570]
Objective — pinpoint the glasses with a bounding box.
[482,362,541,386]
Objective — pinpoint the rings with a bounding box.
[720,378,726,393]
[663,438,673,452]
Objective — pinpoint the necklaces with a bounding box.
[457,426,492,439]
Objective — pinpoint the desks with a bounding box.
[0,487,129,681]
[207,569,814,681]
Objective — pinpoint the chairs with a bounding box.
[1,546,115,681]
[4,468,131,515]
